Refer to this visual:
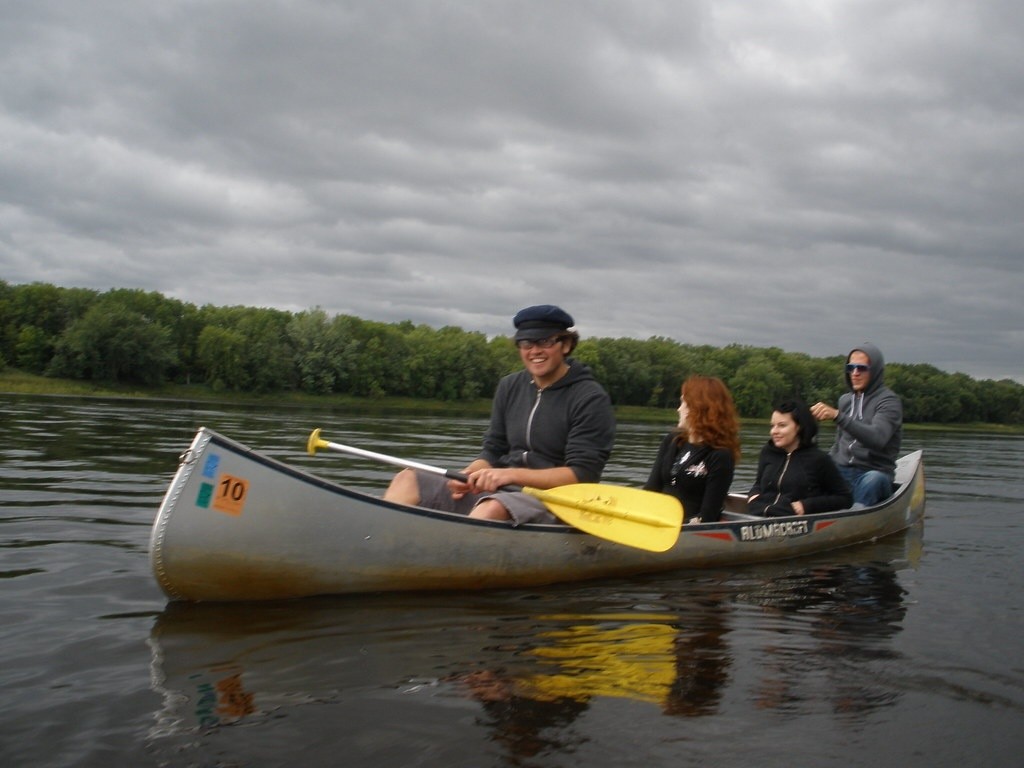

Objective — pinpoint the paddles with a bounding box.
[304,426,686,555]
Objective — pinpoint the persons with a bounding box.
[810,340,903,511]
[642,373,742,523]
[746,399,854,517]
[383,304,616,527]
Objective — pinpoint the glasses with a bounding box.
[517,335,566,349]
[846,364,869,373]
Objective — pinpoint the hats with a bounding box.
[514,305,573,339]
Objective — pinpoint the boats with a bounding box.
[146,425,925,605]
